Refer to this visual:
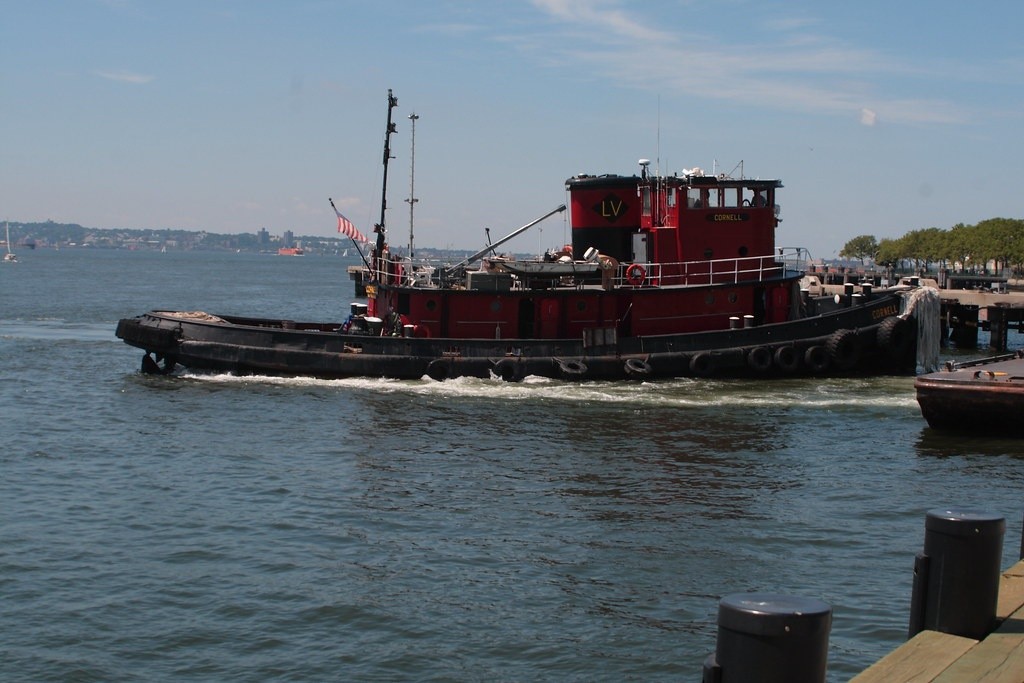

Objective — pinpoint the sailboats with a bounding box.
[4,220,18,263]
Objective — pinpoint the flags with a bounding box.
[336,208,369,243]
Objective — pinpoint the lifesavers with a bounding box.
[626,263,646,285]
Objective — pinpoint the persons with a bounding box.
[750,190,768,207]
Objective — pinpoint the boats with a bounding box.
[113,87,939,383]
[912,346,1023,439]
